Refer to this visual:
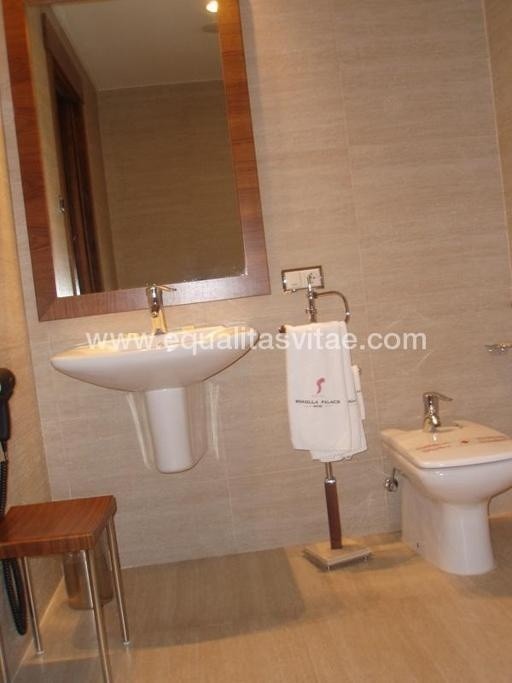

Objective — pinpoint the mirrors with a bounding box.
[0,0,270,324]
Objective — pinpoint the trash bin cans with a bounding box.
[62,527,114,609]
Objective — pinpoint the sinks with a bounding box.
[51,324,259,387]
[380,421,512,505]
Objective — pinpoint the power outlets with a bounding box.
[301,269,325,290]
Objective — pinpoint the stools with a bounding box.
[1,495,130,683]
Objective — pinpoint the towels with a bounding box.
[285,322,368,464]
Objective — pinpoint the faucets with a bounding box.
[146,283,177,335]
[423,389,452,430]
